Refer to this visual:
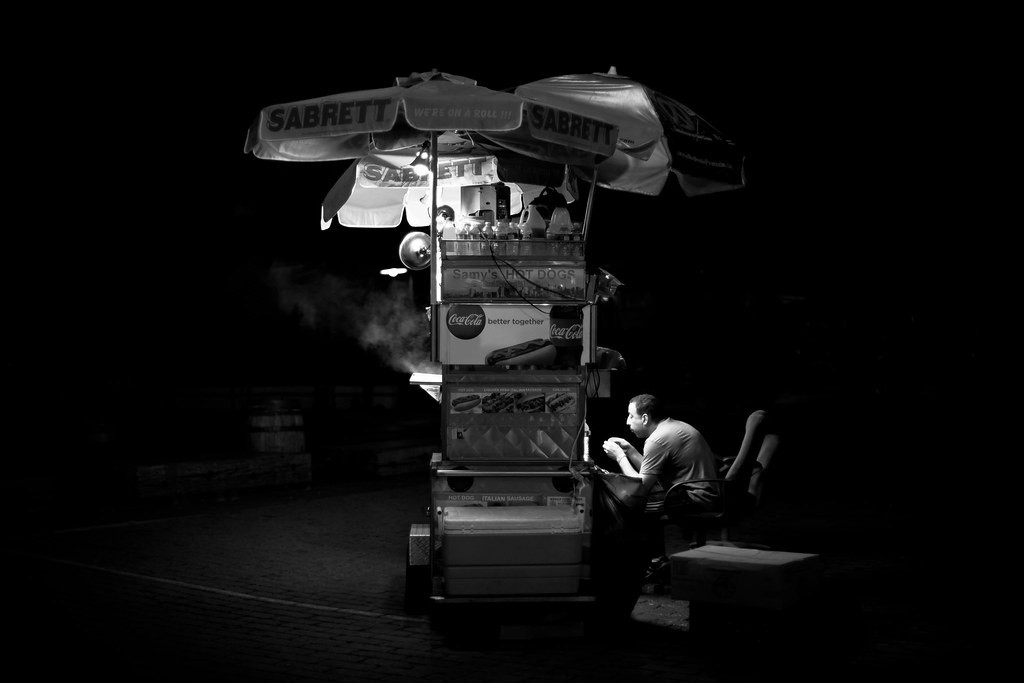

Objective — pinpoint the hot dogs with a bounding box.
[517,392,546,412]
[548,393,574,412]
[451,395,481,411]
[485,337,556,365]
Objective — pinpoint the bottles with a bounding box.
[497,205,506,219]
[550,201,572,233]
[481,222,494,255]
[547,222,555,256]
[506,222,518,256]
[458,224,468,256]
[519,204,546,238]
[469,224,481,255]
[494,222,506,255]
[560,223,571,257]
[550,305,583,371]
[520,223,533,256]
[444,217,457,255]
[573,223,582,256]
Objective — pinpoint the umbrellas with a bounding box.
[244,62,746,368]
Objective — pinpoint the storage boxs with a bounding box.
[667,543,822,608]
[441,507,584,595]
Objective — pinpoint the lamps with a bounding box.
[399,232,431,271]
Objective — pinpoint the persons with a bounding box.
[564,393,722,636]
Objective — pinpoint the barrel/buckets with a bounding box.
[250,404,305,454]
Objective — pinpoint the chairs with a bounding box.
[642,409,780,596]
